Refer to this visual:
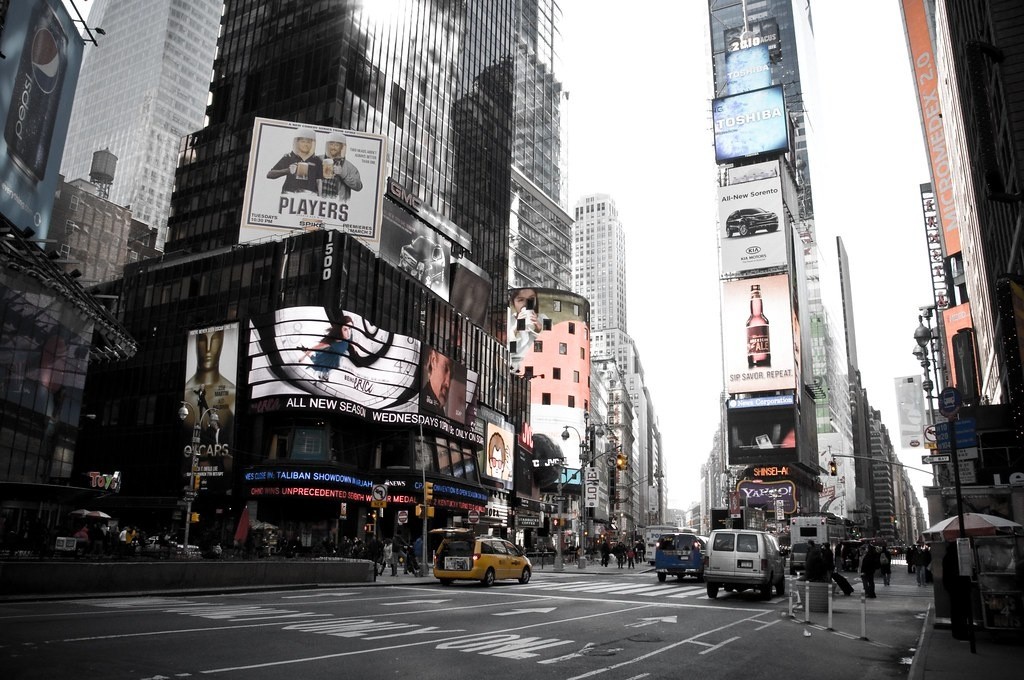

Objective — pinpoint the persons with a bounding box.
[576,539,646,569]
[508,289,552,370]
[343,533,423,576]
[298,315,355,379]
[72,521,179,556]
[798,534,931,598]
[421,349,455,419]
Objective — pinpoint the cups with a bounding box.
[323,159,336,179]
[292,162,309,181]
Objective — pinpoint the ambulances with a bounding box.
[429,526,533,588]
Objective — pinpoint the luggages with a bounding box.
[832,571,855,597]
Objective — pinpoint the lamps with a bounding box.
[90,286,101,295]
[70,269,82,279]
[22,226,35,239]
[47,249,61,260]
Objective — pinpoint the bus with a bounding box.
[643,525,697,567]
[789,516,861,573]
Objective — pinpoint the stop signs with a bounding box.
[398,512,408,522]
[469,511,479,523]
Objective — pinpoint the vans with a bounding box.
[706,529,821,600]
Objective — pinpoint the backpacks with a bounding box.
[880,551,889,565]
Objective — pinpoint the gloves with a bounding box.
[289,162,298,174]
[334,165,343,175]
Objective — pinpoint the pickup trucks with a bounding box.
[654,534,709,582]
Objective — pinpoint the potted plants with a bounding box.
[795,547,838,611]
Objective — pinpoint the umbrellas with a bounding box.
[86,510,111,520]
[921,512,1022,542]
[252,521,279,545]
[234,508,249,543]
[68,508,90,518]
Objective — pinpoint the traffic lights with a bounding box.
[830,461,836,476]
[617,455,622,470]
[341,503,347,516]
[623,455,628,470]
[416,503,435,518]
[364,524,375,532]
[426,481,434,502]
[553,518,567,527]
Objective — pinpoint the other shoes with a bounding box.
[865,595,876,598]
[918,582,927,586]
[627,567,630,569]
[618,566,624,569]
[633,567,635,569]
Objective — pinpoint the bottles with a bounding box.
[746,284,771,369]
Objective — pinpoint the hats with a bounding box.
[324,133,347,158]
[292,127,316,156]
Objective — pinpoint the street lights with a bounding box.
[912,303,948,487]
[175,400,222,550]
[561,422,605,556]
